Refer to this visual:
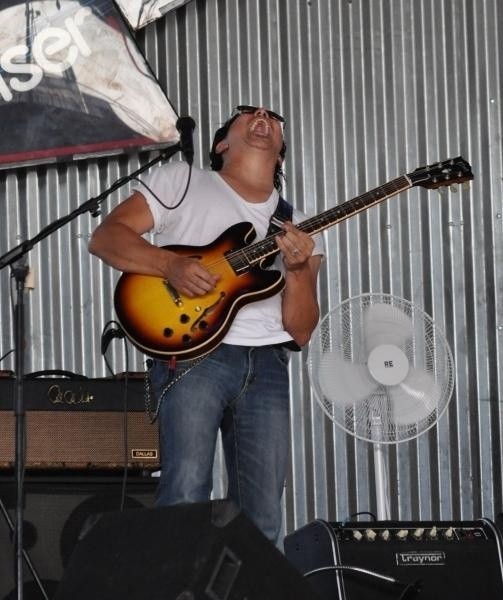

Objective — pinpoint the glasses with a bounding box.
[237,105,285,130]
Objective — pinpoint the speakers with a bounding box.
[55,497,321,600]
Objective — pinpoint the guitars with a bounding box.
[114,157,471,363]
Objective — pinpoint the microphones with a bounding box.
[175,115,196,167]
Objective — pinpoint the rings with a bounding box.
[292,250,300,255]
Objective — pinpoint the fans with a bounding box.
[307,292,455,522]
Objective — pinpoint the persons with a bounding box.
[88,104,325,551]
